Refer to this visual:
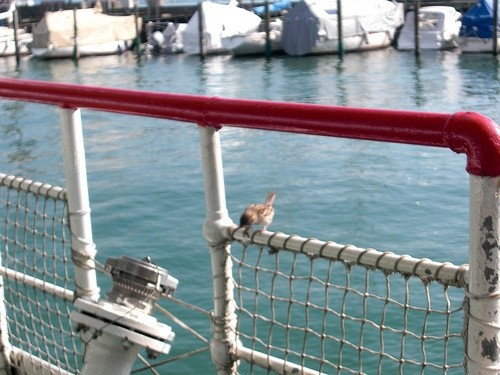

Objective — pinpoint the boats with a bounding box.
[153,23,187,54]
[220,16,284,58]
[182,1,264,55]
[32,9,142,58]
[461,0,500,53]
[398,5,463,51]
[0,26,33,56]
[281,0,404,55]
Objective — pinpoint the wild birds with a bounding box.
[239,192,275,237]
